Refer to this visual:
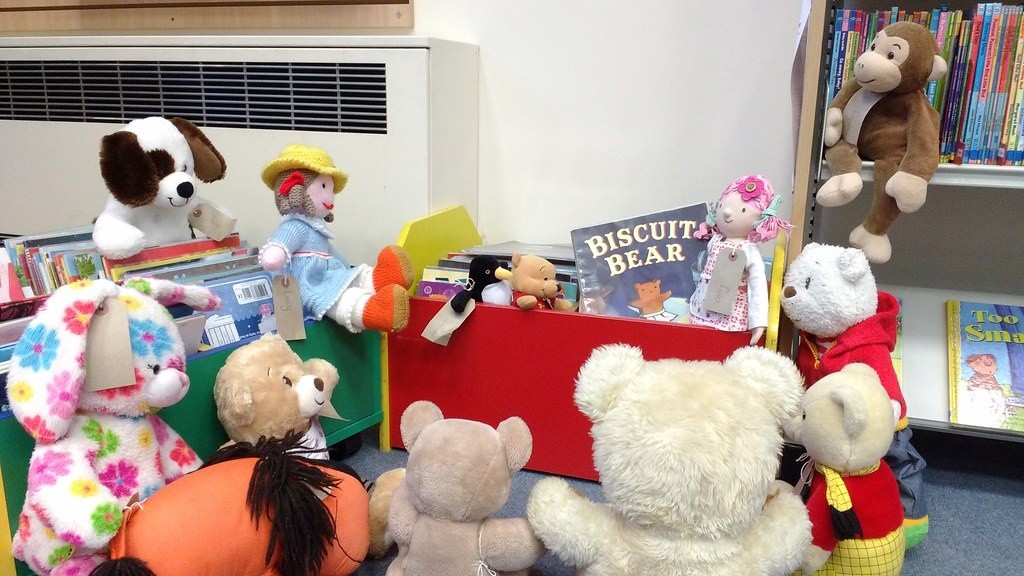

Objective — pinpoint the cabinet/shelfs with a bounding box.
[779,0,1024,454]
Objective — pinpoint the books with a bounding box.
[5,222,279,353]
[811,1,1024,435]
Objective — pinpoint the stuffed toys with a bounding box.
[259,146,415,332]
[92,114,229,260]
[817,22,954,263]
[6,176,931,576]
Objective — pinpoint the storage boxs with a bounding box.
[384,217,787,482]
[0,306,382,576]
